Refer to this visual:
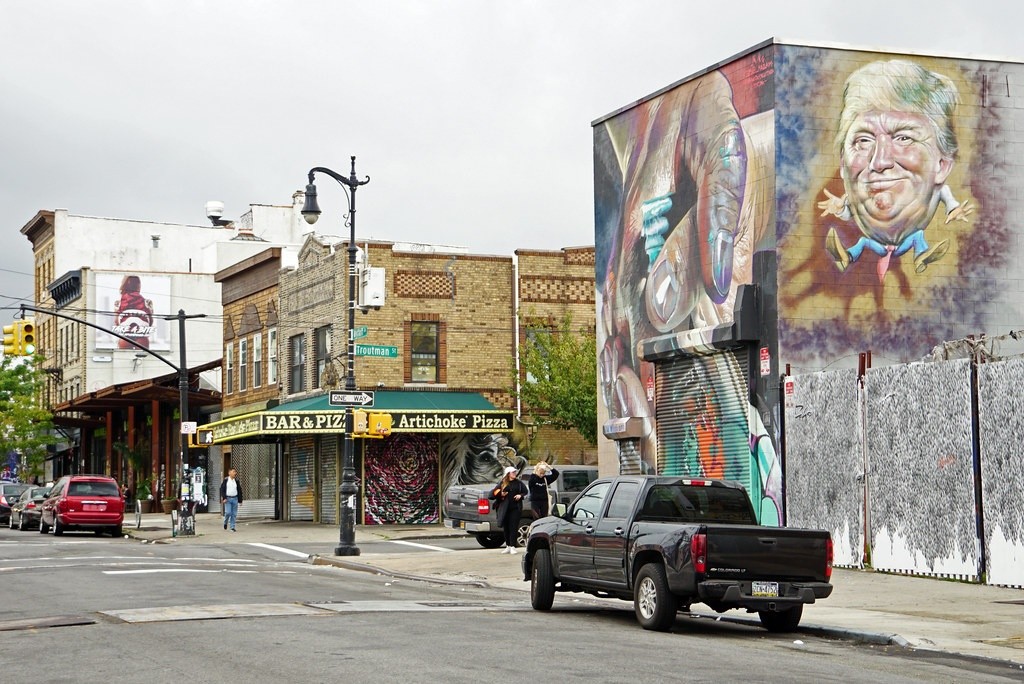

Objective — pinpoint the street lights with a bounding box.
[300,155,370,556]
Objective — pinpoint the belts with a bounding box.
[226,495,238,498]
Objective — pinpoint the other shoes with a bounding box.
[231,528,236,532]
[224,523,227,530]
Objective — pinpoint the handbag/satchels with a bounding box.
[544,477,555,517]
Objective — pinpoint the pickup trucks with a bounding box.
[444,464,834,632]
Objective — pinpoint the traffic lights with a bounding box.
[4,320,38,356]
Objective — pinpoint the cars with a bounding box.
[0,475,125,537]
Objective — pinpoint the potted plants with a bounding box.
[136,480,153,513]
[160,497,178,514]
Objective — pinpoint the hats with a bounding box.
[501,466,517,481]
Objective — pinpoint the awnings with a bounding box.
[187,390,516,448]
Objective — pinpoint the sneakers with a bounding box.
[509,546,517,554]
[501,546,511,554]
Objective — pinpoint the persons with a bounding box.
[488,465,528,555]
[528,460,560,522]
[219,468,243,533]
[122,483,131,498]
